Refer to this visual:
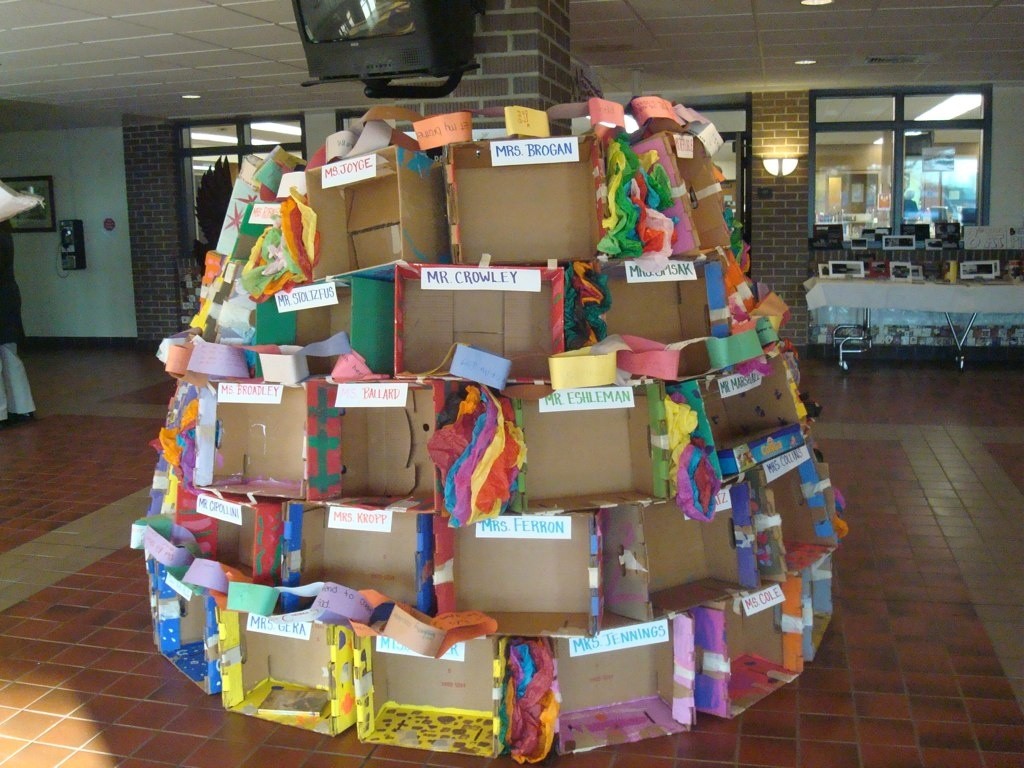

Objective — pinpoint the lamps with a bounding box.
[761,157,800,181]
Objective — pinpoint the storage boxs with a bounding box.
[144,128,840,759]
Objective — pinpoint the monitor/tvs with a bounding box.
[292,0,480,78]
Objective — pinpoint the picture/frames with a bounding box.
[0,174,57,234]
[924,238,943,251]
[889,261,913,283]
[855,254,876,272]
[868,261,891,279]
[882,235,917,251]
[964,260,1000,276]
[850,237,869,250]
[827,261,865,279]
[959,262,995,280]
[817,263,830,279]
[911,266,923,281]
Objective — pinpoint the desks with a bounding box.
[802,275,1024,375]
